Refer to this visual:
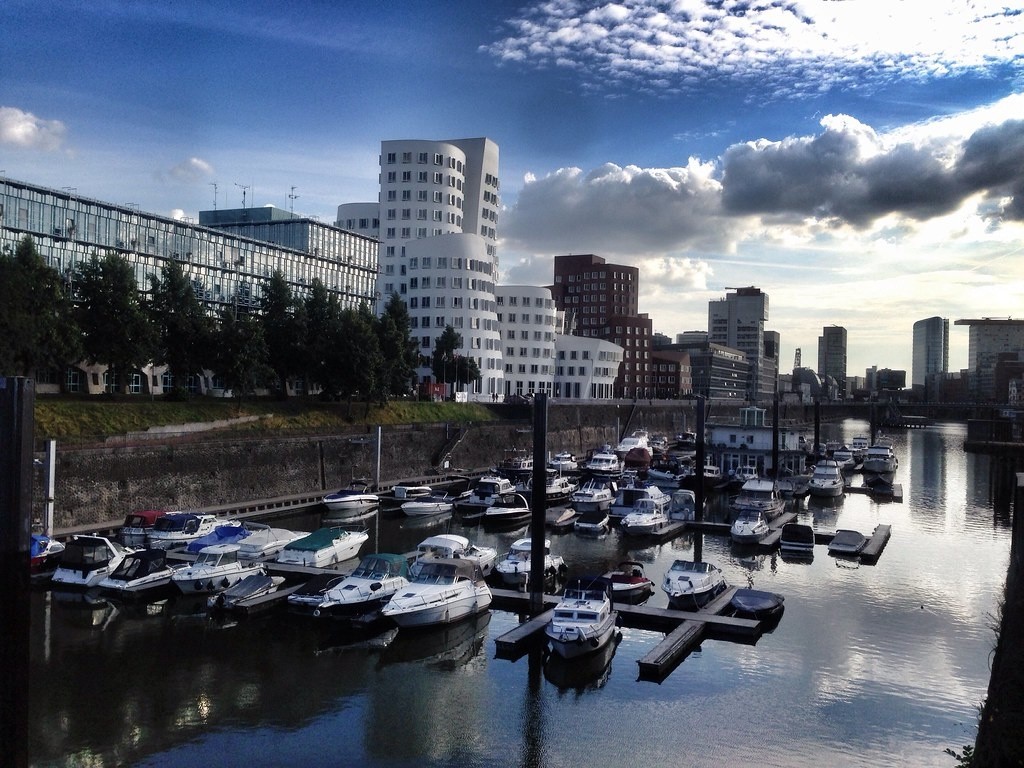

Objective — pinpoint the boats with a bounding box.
[827,529,868,555]
[383,424,900,537]
[732,588,786,625]
[730,508,772,543]
[595,560,656,604]
[382,612,493,664]
[315,553,409,616]
[114,508,370,569]
[50,535,145,587]
[173,543,266,593]
[96,546,196,596]
[379,556,495,626]
[778,522,816,561]
[542,632,624,676]
[414,533,499,573]
[203,574,301,617]
[303,597,388,651]
[322,478,380,509]
[544,573,624,651]
[496,537,568,588]
[661,558,726,612]
[29,534,65,570]
